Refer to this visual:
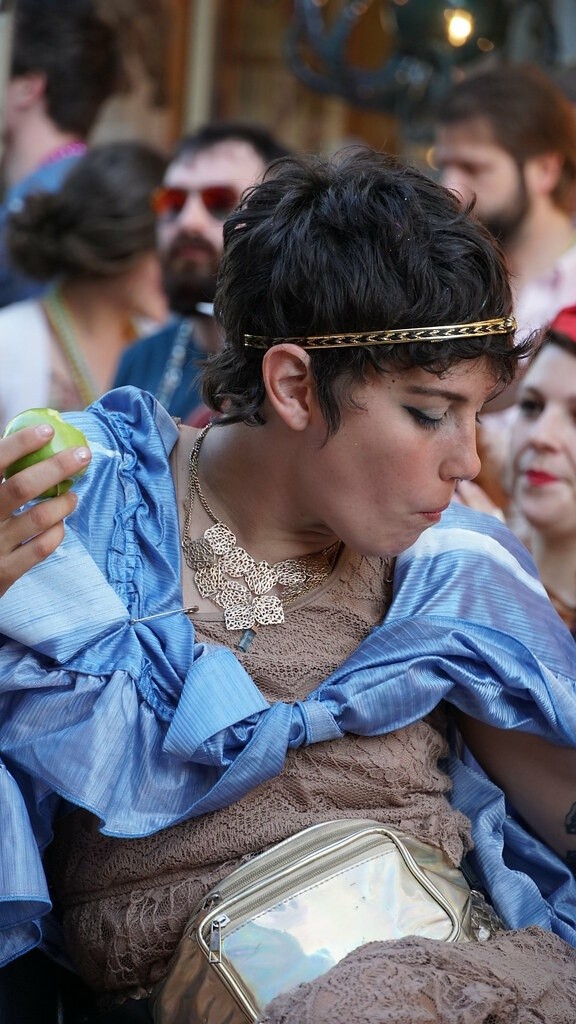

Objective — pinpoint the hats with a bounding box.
[550,305,576,349]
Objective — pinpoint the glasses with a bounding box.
[150,183,239,221]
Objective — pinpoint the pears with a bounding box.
[1,408,92,497]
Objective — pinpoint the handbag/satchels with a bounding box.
[138,816,474,1022]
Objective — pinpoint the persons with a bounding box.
[0,0,123,299]
[434,66,576,520]
[2,138,171,428]
[115,122,296,423]
[2,144,576,1024]
[506,303,576,638]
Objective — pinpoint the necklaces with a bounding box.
[180,419,346,629]
